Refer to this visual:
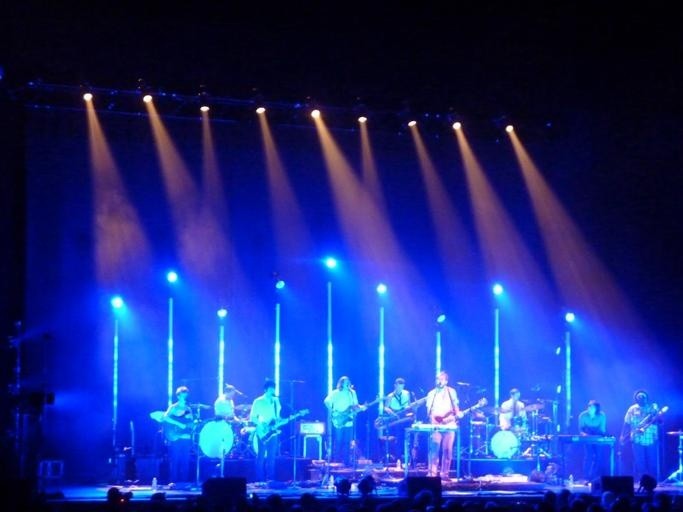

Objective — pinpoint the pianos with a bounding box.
[558,435,615,444]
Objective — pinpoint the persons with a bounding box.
[578,400,605,478]
[427,371,464,481]
[619,391,664,483]
[164,386,198,489]
[471,387,527,442]
[215,380,295,484]
[384,378,415,464]
[324,375,366,469]
[105,487,683,512]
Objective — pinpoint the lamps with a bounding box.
[136,82,155,107]
[253,87,269,116]
[450,112,465,132]
[306,96,324,121]
[405,113,419,129]
[79,85,96,104]
[354,106,370,124]
[194,91,213,113]
[501,116,515,136]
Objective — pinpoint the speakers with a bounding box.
[591,475,635,495]
[395,475,442,499]
[202,476,248,497]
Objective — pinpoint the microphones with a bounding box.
[346,383,355,388]
[410,392,416,397]
[272,394,277,397]
[456,381,470,386]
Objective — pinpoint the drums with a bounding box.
[489,426,518,459]
[192,417,239,459]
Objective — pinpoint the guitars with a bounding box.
[164,416,194,442]
[388,397,426,427]
[259,409,309,444]
[332,399,378,428]
[435,398,488,425]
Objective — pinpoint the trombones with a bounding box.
[620,403,669,445]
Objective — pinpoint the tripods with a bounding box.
[461,416,490,456]
[522,411,551,458]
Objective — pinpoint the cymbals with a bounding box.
[234,404,252,410]
[488,407,511,414]
[190,404,211,409]
[518,405,544,411]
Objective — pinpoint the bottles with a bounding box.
[568,474,573,486]
[152,477,158,494]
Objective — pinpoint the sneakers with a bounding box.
[254,481,267,486]
[185,485,198,491]
[169,483,176,490]
[441,477,452,483]
